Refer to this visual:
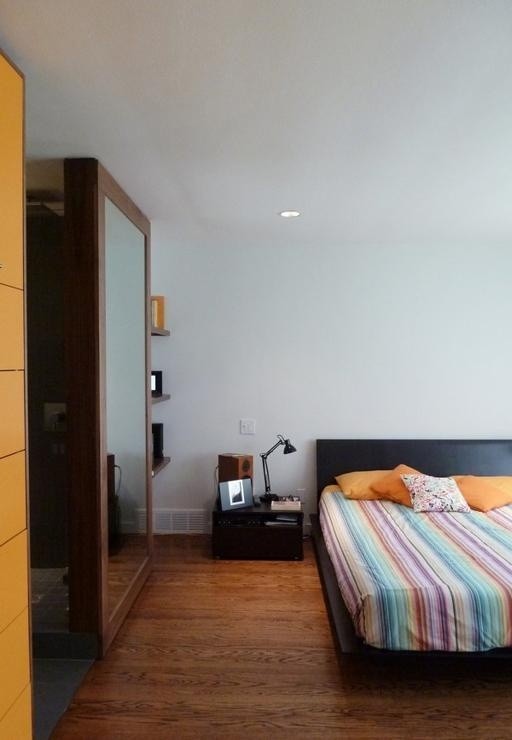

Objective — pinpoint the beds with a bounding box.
[306,439,512,676]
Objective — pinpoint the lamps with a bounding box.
[260,433,296,504]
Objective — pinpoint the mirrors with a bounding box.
[63,158,155,660]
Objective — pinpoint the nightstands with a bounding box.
[212,495,304,562]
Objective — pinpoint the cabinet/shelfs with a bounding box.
[152,327,171,476]
[2,50,35,738]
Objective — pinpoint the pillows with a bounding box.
[334,469,395,501]
[399,473,471,514]
[370,464,422,508]
[453,474,512,511]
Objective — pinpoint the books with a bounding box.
[271,495,302,510]
[264,515,298,526]
